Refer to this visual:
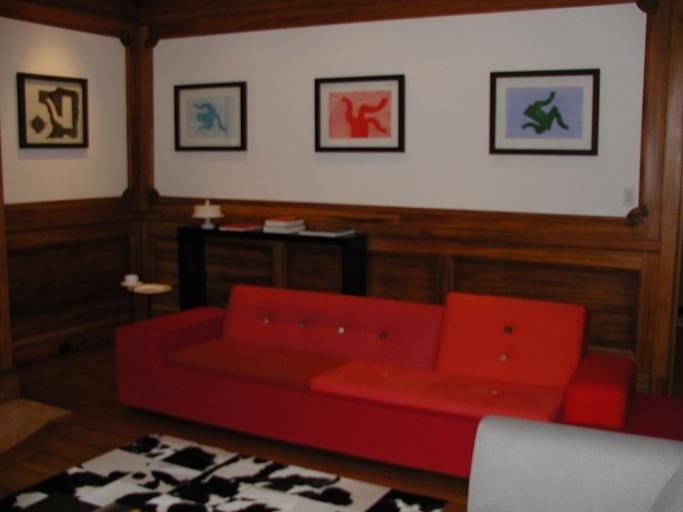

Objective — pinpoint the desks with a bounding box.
[176,223,369,309]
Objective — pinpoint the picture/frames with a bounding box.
[311,73,407,154]
[17,71,89,149]
[487,70,601,155]
[171,80,249,152]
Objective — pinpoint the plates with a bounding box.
[120,282,172,294]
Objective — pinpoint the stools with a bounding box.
[131,283,174,318]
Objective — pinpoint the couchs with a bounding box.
[115,284,636,482]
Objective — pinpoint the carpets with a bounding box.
[1,434,450,511]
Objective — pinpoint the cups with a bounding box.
[124,275,138,284]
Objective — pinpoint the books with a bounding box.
[298,230,356,239]
[218,222,261,233]
[262,216,307,235]
